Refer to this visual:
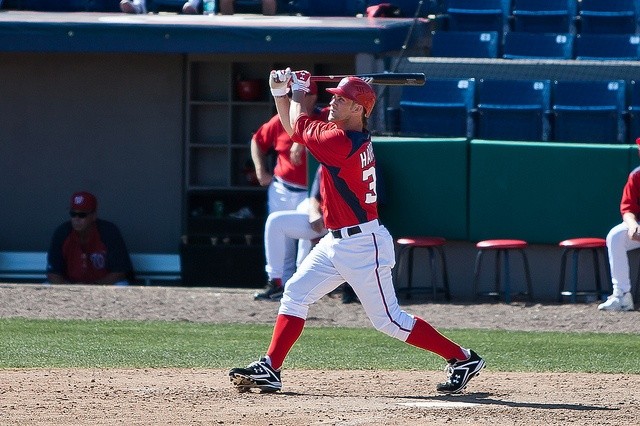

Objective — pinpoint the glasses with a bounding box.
[70,210,95,217]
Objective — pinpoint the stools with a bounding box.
[475,240,535,307]
[394,237,451,302]
[558,237,614,307]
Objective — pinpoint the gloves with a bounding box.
[290,69,311,93]
[269,66,291,95]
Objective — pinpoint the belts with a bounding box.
[273,176,310,194]
[327,217,383,239]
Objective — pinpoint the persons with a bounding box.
[596,137,640,310]
[253,167,384,303]
[228,65,486,395]
[120,0,201,14]
[45,191,133,285]
[249,80,330,288]
[219,0,276,15]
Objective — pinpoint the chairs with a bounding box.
[401,0,640,142]
[0,253,182,289]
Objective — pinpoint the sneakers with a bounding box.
[597,292,636,311]
[253,282,285,302]
[229,355,282,391]
[436,347,487,393]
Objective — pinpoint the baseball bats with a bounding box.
[274,73,426,85]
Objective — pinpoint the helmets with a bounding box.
[325,75,376,118]
[72,191,97,208]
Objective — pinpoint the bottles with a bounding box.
[202,0,215,16]
